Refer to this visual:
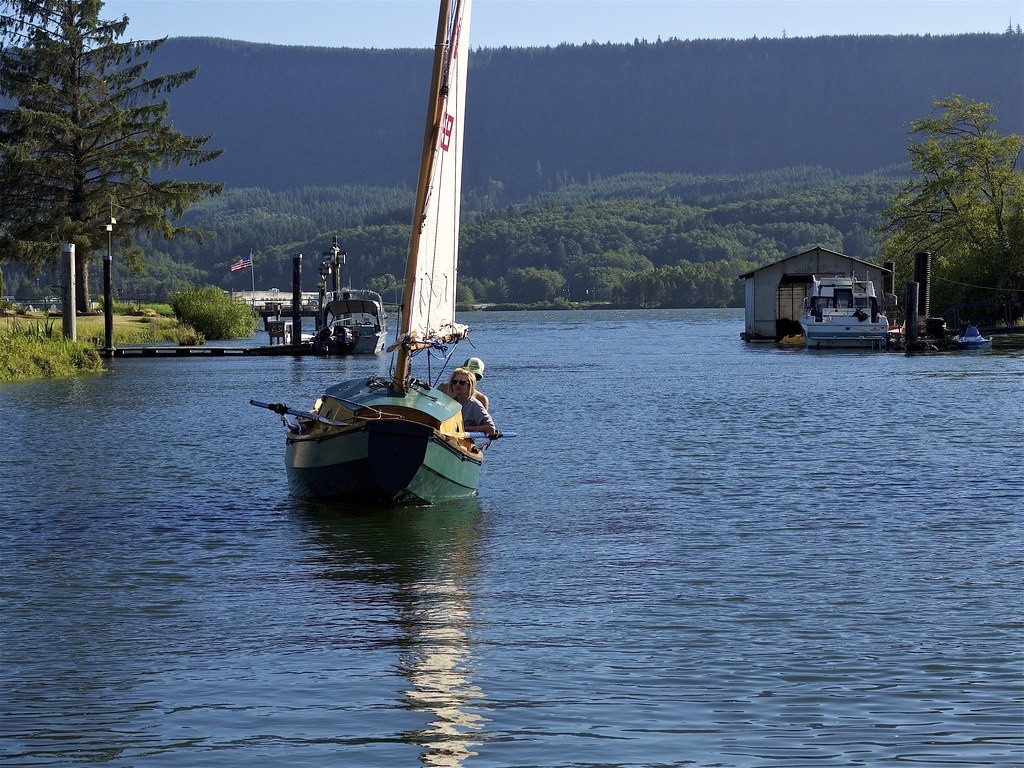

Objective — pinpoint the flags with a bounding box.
[231,253,251,272]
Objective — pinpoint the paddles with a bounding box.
[440,431,517,438]
[250,399,352,426]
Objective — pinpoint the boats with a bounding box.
[779,333,806,348]
[951,325,993,352]
[317,275,389,355]
[797,270,889,350]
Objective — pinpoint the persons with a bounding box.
[437,357,496,443]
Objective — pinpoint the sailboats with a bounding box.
[247,1,519,509]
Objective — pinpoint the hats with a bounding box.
[461,358,485,381]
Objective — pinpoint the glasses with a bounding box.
[452,379,468,386]
